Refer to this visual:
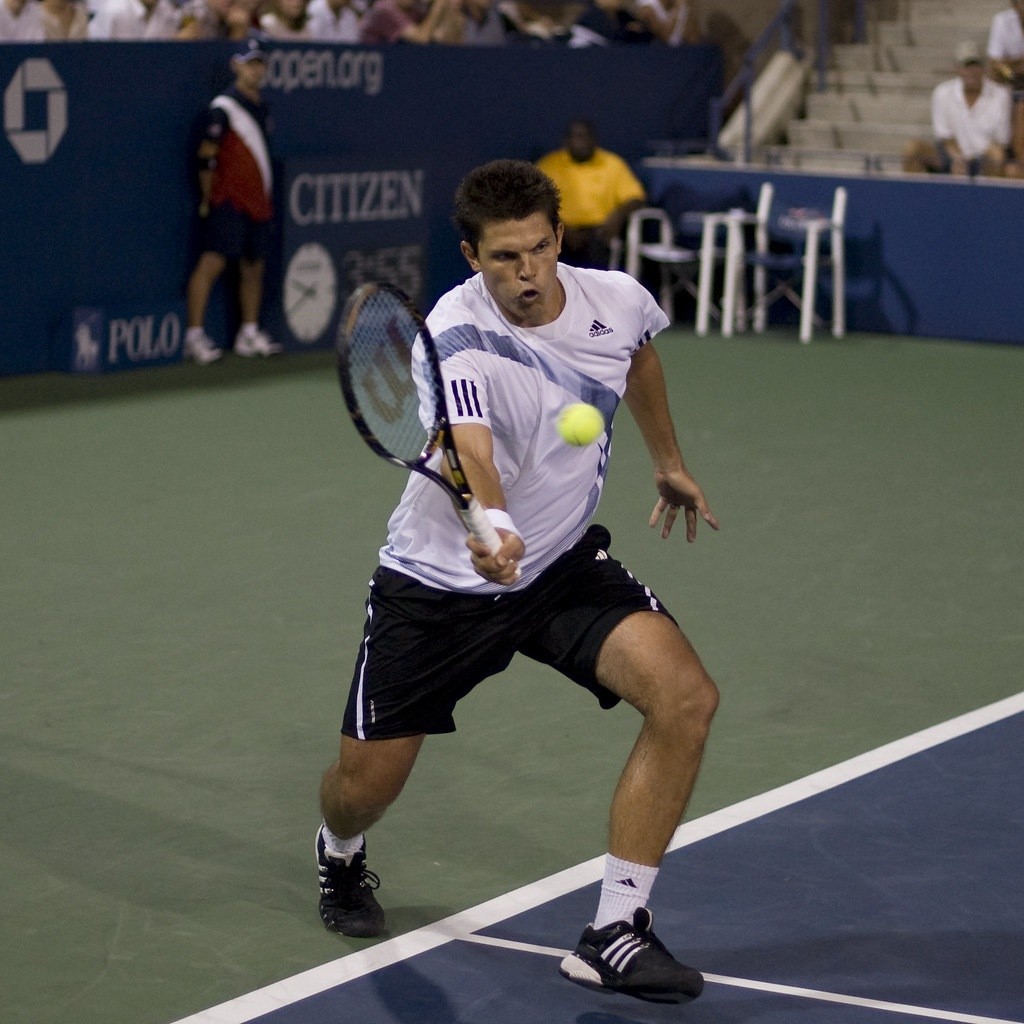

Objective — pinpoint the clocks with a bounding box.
[286,242,338,344]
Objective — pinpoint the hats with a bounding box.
[237,38,267,63]
[957,38,982,64]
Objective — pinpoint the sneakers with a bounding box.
[314,824,385,937]
[560,907,704,1004]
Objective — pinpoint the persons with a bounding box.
[0,0,1024,366]
[313,162,726,1008]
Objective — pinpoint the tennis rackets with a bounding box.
[336,281,521,580]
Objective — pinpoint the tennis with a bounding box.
[556,401,605,447]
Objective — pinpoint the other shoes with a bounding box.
[181,337,223,364]
[235,332,281,356]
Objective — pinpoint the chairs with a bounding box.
[577,181,849,338]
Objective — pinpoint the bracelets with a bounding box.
[482,508,521,542]
